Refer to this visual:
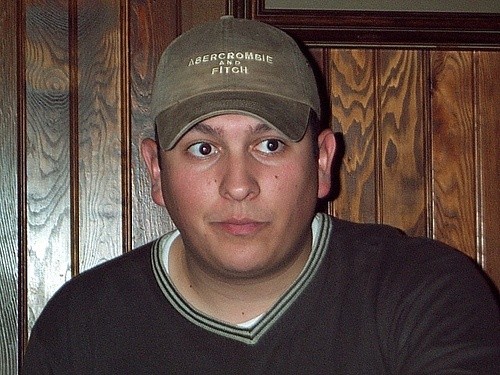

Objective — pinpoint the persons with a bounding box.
[16,14,500,375]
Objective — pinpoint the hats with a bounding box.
[151,15,325,151]
